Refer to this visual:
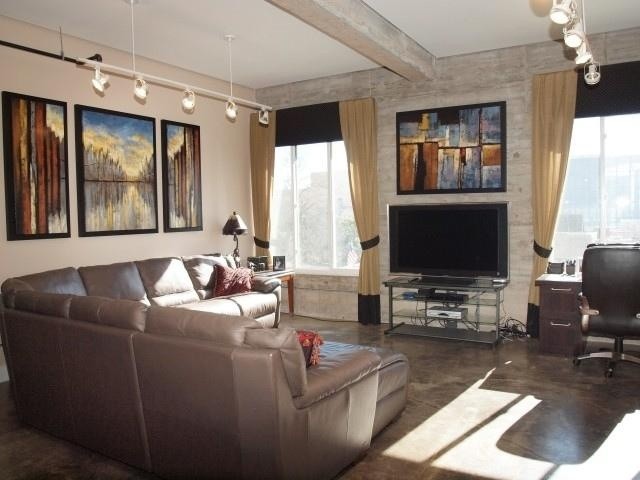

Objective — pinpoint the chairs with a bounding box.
[572,243,640,377]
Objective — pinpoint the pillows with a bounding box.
[295,328,324,368]
[213,264,256,297]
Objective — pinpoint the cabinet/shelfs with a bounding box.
[537,273,583,354]
[381,276,510,348]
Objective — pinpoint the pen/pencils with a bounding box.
[565,259,576,266]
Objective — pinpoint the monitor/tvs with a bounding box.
[389,203,507,286]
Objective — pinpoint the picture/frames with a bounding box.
[395,101,507,195]
[273,255,286,272]
[247,257,259,271]
[2,91,72,240]
[160,118,203,233]
[260,256,271,271]
[73,105,159,237]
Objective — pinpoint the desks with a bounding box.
[244,269,295,318]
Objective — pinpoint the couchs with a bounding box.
[1,252,409,479]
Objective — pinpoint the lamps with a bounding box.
[573,38,593,66]
[560,18,587,50]
[180,88,196,114]
[583,59,602,87]
[224,97,238,121]
[258,107,270,126]
[87,65,111,95]
[132,74,150,102]
[548,0,577,26]
[222,210,249,269]
